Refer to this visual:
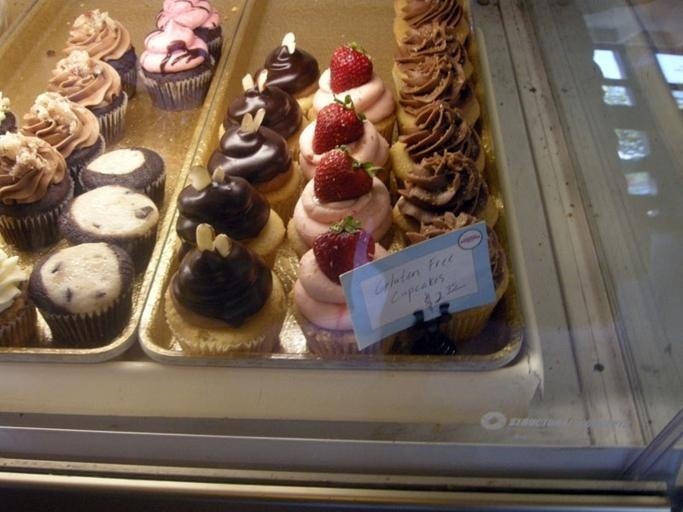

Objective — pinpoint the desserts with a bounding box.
[161,0,509,356]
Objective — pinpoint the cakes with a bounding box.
[49,50,130,143]
[154,0,237,60]
[137,20,217,112]
[0,91,18,134]
[0,244,36,351]
[0,131,75,247]
[65,8,142,100]
[25,91,103,167]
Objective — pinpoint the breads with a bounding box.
[76,147,166,207]
[29,239,135,349]
[58,183,160,273]
